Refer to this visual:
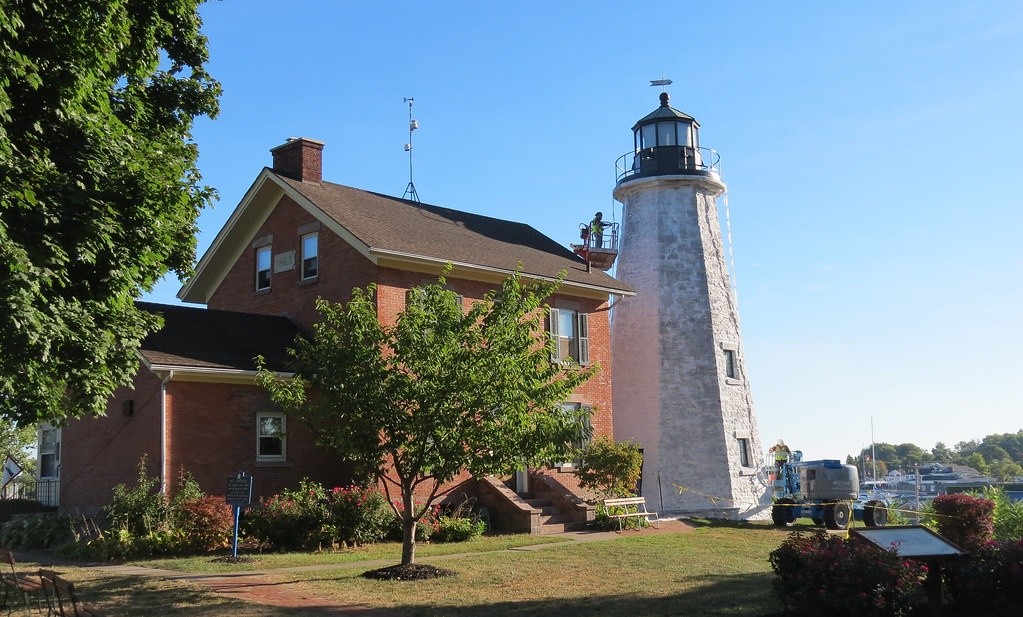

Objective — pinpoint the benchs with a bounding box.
[0,545,43,617]
[39,565,109,617]
[604,495,657,533]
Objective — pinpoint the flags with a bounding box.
[1,455,23,499]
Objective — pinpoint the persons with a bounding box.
[769,438,792,480]
[593,212,612,248]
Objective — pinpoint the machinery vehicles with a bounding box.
[762,450,888,531]
